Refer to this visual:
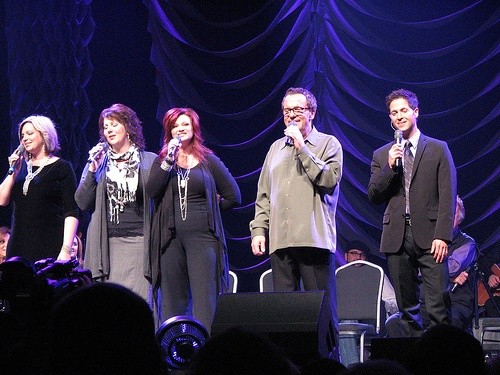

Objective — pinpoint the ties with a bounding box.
[404,141,414,214]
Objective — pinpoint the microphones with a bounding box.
[8,145,25,175]
[393,130,403,168]
[168,136,181,157]
[285,120,297,147]
[450,266,471,293]
[88,142,109,163]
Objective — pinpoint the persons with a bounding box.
[0,116,79,263]
[478,249,500,318]
[0,282,500,375]
[74,104,159,307]
[344,239,400,319]
[0,226,11,264]
[71,234,82,264]
[145,108,242,334]
[250,86,344,336]
[368,88,458,328]
[384,196,478,338]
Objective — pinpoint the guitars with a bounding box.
[476,253,500,306]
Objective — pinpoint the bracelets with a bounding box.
[160,160,173,172]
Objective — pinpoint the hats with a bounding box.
[346,240,369,252]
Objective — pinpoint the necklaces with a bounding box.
[172,156,194,220]
[23,154,53,196]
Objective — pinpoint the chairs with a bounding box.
[335,260,385,364]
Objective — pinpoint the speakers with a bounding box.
[209,292,341,368]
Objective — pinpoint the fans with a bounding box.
[155,317,210,370]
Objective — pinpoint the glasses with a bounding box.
[347,251,367,260]
[281,106,309,116]
